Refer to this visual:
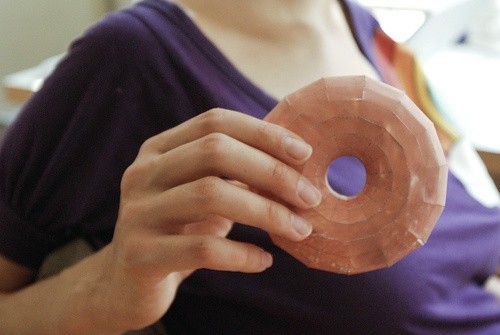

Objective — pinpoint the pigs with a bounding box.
[250,74,449,274]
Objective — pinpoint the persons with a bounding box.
[0,0,500,334]
